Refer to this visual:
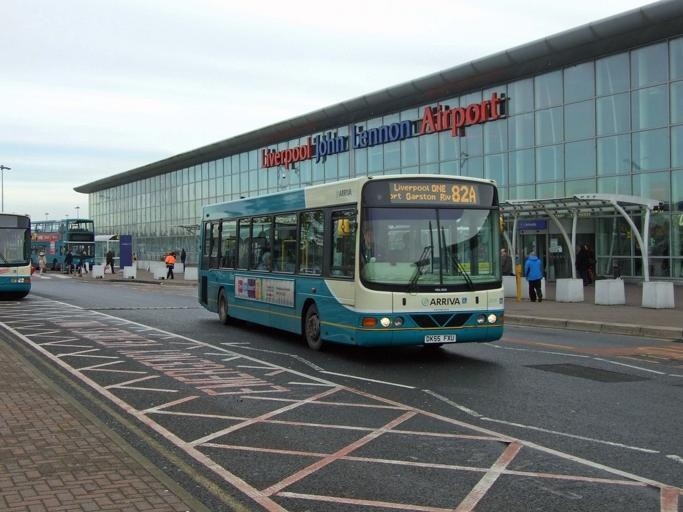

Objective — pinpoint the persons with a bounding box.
[37,251,47,275]
[257,252,277,271]
[65,249,73,275]
[500,248,513,276]
[524,251,544,301]
[78,252,88,274]
[579,242,594,286]
[259,231,267,239]
[353,230,390,266]
[164,253,176,279]
[105,249,116,274]
[308,213,323,265]
[132,253,137,263]
[180,249,187,271]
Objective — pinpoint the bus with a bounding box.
[30,219,94,273]
[0,214,32,301]
[198,174,504,352]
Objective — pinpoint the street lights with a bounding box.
[1,166,11,212]
